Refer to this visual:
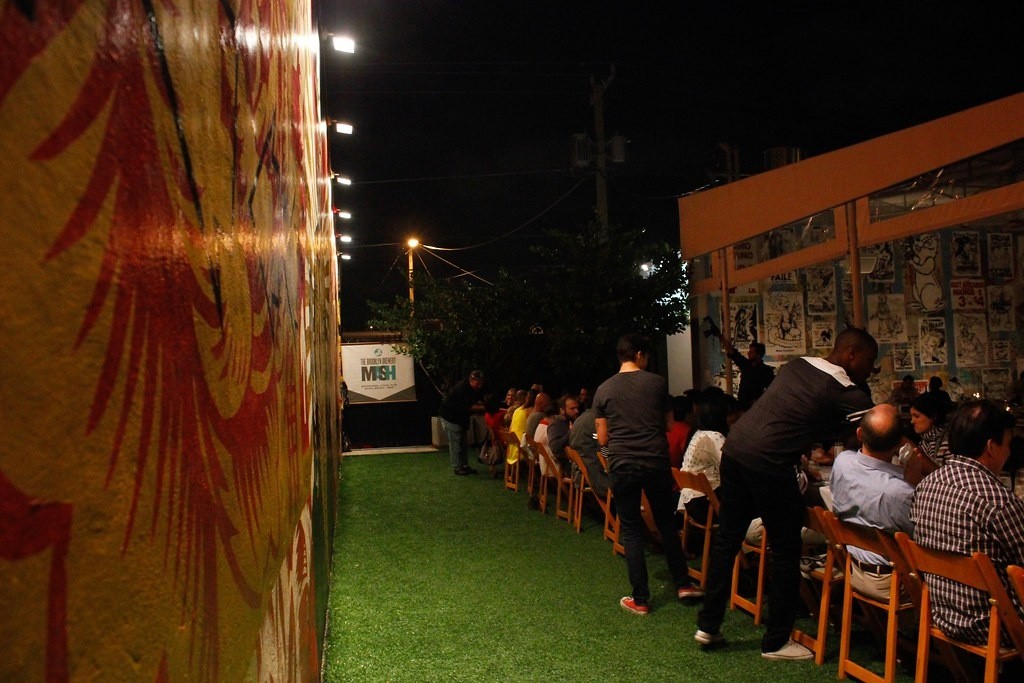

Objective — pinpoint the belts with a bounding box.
[851,555,892,574]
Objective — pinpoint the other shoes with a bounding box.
[620,597,648,617]
[467,468,477,473]
[679,585,705,598]
[694,630,723,644]
[762,640,814,661]
[455,470,468,475]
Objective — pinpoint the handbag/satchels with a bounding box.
[478,429,502,465]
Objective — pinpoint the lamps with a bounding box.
[326,115,354,134]
[333,207,351,219]
[331,172,351,185]
[319,28,355,53]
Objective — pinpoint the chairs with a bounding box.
[729,524,768,627]
[529,439,568,515]
[564,446,593,534]
[612,489,661,557]
[570,449,615,540]
[485,424,499,472]
[671,467,721,591]
[498,430,529,494]
[823,510,922,683]
[523,431,539,495]
[791,506,883,667]
[555,465,575,525]
[894,531,1024,683]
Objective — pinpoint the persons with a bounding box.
[694,325,879,662]
[592,334,704,614]
[437,369,485,477]
[707,316,775,403]
[478,382,1024,683]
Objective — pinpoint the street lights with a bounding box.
[406,238,422,319]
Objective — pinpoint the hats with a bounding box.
[471,371,486,384]
